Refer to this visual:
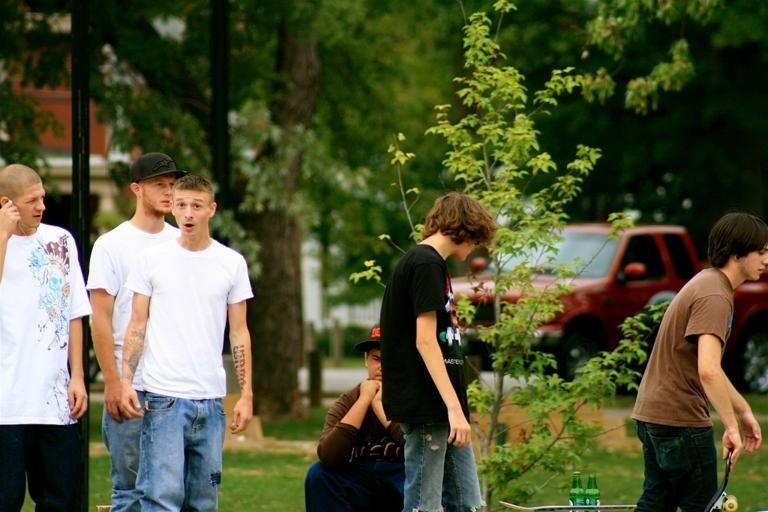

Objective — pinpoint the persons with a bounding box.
[305,326,406,512]
[1,162,93,512]
[379,191,498,511]
[631,214,768,512]
[118,174,255,512]
[86,152,189,512]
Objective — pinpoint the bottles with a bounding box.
[569,471,600,512]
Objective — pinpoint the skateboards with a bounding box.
[703,453,739,512]
[498,501,636,512]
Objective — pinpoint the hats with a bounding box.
[355,327,382,350]
[131,152,190,183]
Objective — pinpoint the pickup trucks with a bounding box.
[449,225,768,393]
